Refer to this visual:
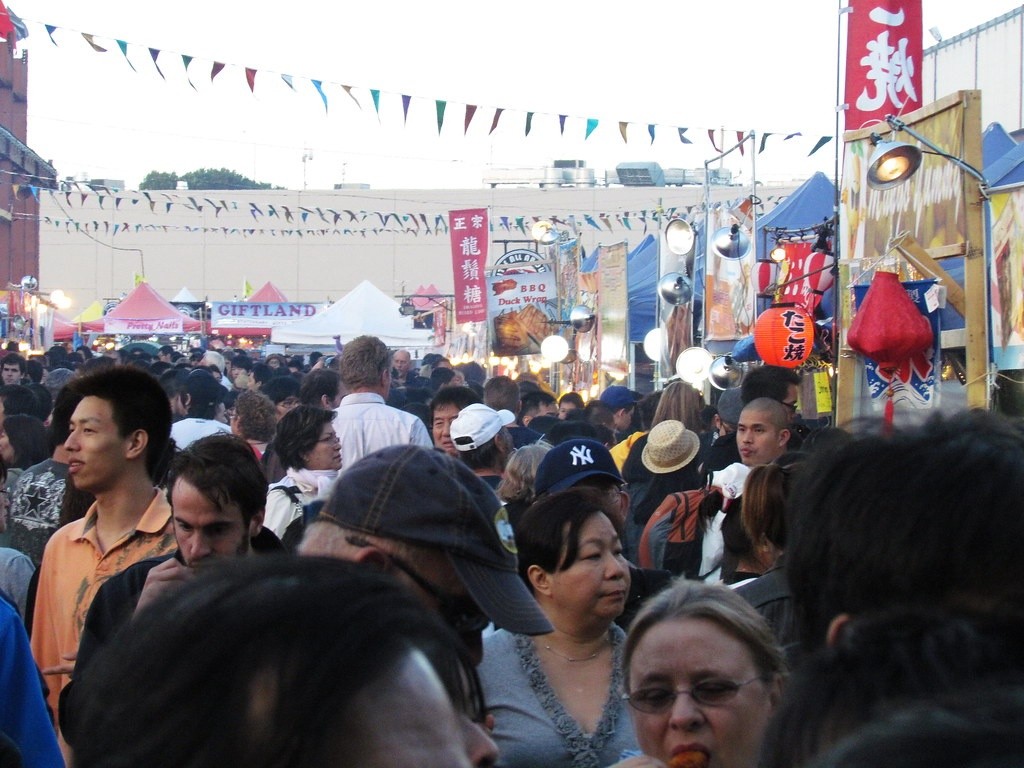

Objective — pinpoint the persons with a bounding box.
[0,336,1024,768]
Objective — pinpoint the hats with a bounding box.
[641,420,700,474]
[321,444,555,636]
[601,385,643,408]
[535,439,627,496]
[450,403,515,451]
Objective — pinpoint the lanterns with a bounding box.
[804,248,835,294]
[751,259,781,297]
[752,303,814,367]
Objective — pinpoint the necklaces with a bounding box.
[543,641,610,662]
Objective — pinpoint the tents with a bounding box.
[54,282,205,342]
[208,282,314,336]
[271,280,450,350]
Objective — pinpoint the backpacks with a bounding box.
[637,468,724,580]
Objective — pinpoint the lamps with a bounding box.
[2,315,26,330]
[866,112,984,192]
[643,200,751,392]
[10,276,38,290]
[526,304,597,361]
[770,243,785,262]
[399,297,414,314]
[492,222,559,245]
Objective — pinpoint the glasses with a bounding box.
[623,675,765,713]
[319,437,340,447]
[344,536,490,636]
[780,402,797,414]
